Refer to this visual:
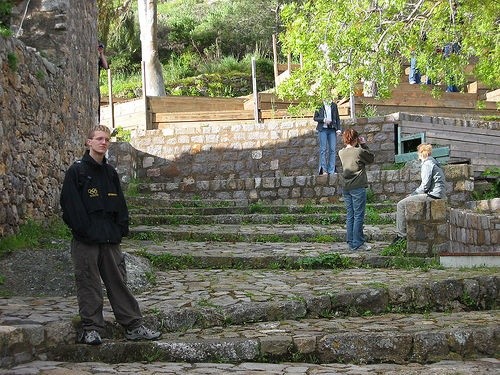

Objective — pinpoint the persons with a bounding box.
[409,23,466,94]
[61,123,164,346]
[337,128,375,252]
[313,99,342,175]
[98,39,110,123]
[388,142,446,247]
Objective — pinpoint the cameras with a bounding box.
[328,120,336,128]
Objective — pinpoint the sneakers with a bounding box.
[126,324,162,341]
[84,329,103,344]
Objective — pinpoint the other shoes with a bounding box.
[389,234,402,246]
[356,243,371,251]
